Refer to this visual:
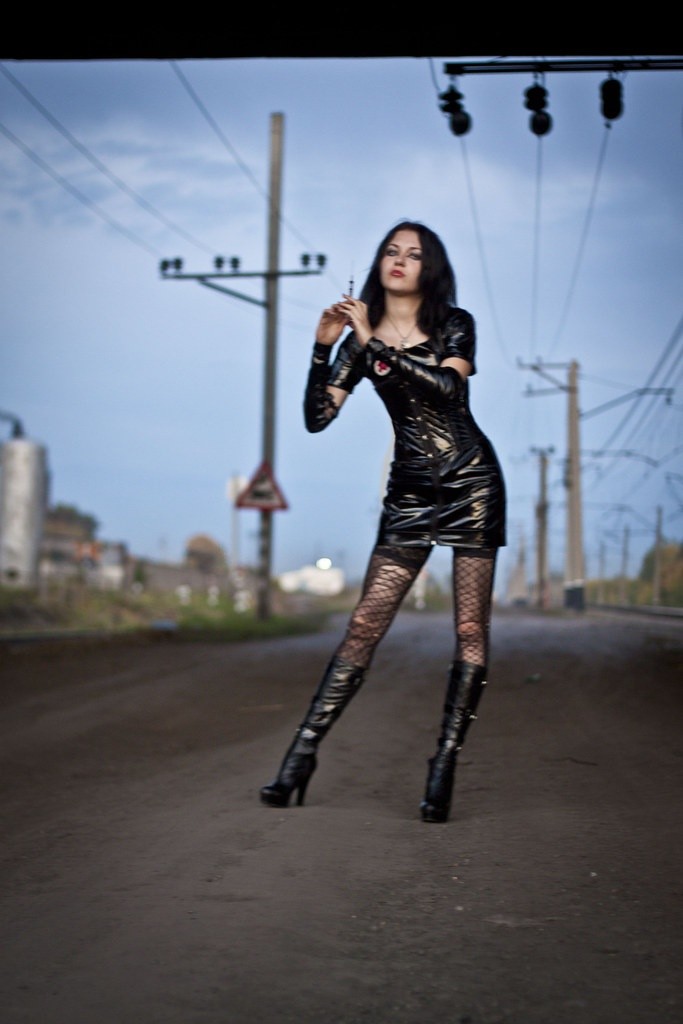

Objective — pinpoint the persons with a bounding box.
[258,222,509,824]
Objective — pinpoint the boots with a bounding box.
[417,658,491,824]
[259,654,371,810]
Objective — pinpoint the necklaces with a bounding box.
[383,315,417,348]
